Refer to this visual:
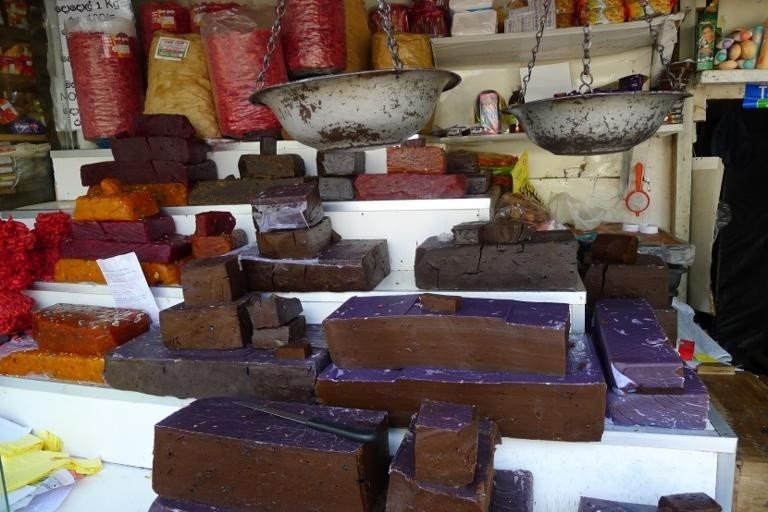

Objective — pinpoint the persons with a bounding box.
[698,24,715,61]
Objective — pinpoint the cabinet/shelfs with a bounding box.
[0,11,767,512]
